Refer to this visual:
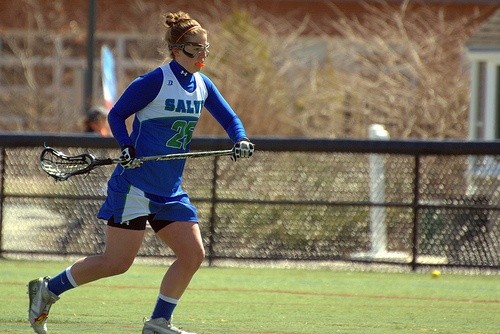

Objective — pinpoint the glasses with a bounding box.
[173,41,210,58]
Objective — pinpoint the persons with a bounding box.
[26,11,254,334]
[57,107,112,256]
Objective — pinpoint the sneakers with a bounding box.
[27,275,60,334]
[142,315,189,334]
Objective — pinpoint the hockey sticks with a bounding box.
[39,144,240,180]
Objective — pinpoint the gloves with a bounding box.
[119,147,143,168]
[230,140,254,162]
